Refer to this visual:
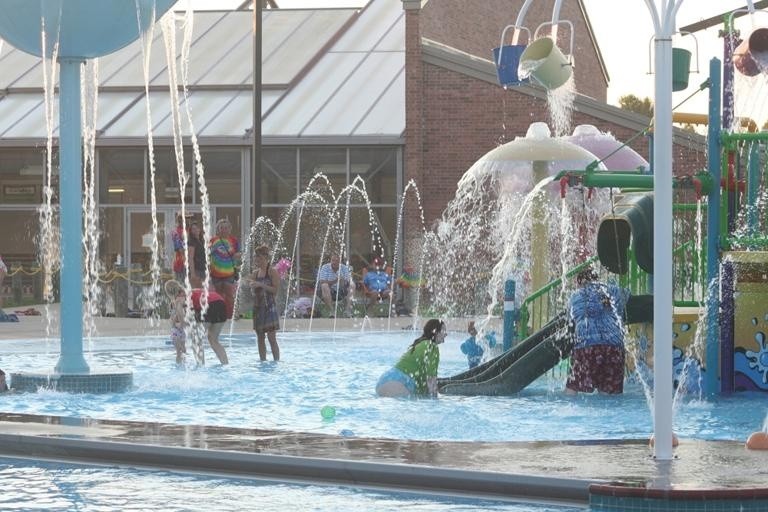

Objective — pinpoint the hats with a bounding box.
[253,246,269,257]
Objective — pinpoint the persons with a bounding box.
[376,317,449,398]
[170,208,193,281]
[170,302,189,365]
[565,266,631,394]
[164,278,229,364]
[185,220,206,290]
[459,320,496,369]
[245,246,283,360]
[0,256,8,308]
[360,256,400,316]
[316,253,357,319]
[208,219,242,319]
[0,370,16,396]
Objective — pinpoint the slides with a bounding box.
[434,294,655,395]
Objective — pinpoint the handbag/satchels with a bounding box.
[253,290,268,309]
[233,267,240,281]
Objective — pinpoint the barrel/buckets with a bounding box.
[672,44,693,92]
[491,25,532,87]
[728,9,767,77]
[518,18,575,94]
